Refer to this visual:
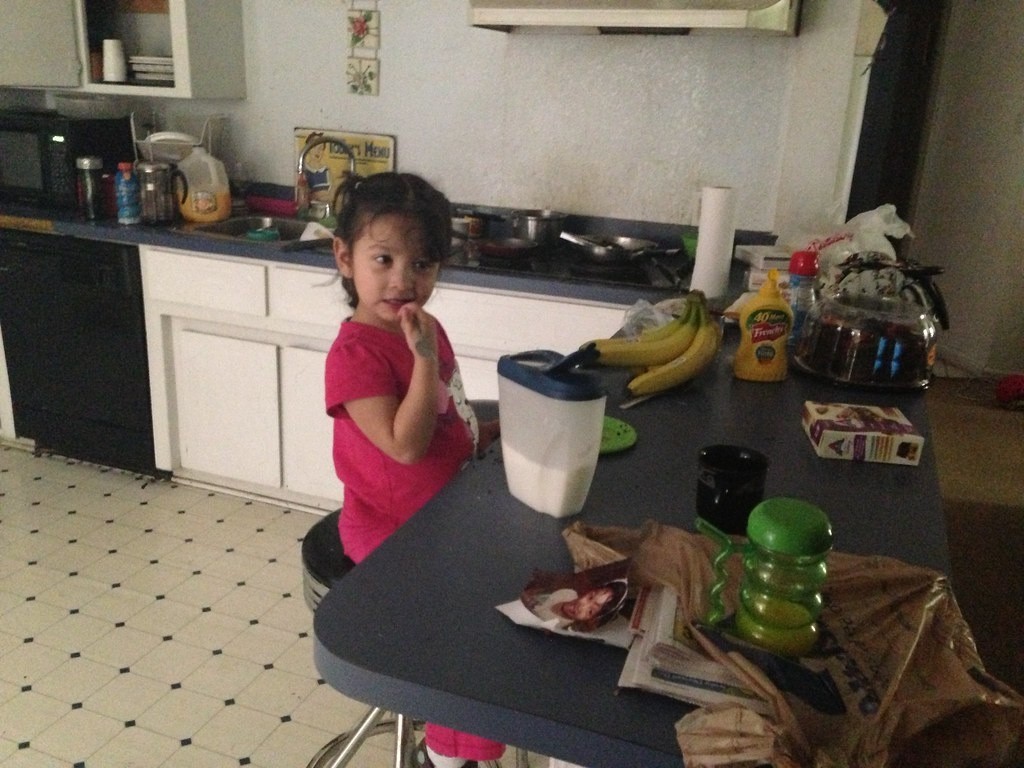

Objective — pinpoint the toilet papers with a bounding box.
[689,185,739,300]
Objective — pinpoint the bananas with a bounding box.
[581,290,722,396]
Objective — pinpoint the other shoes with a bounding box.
[409,738,478,768]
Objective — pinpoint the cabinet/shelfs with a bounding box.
[0,0,245,100]
[0,224,630,518]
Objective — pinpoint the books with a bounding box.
[129,55,175,81]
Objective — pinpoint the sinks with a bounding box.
[190,215,338,251]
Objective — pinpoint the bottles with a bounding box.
[737,497,834,657]
[75,156,108,223]
[113,163,141,225]
[176,143,231,222]
[786,249,820,349]
[733,270,793,383]
[296,178,310,220]
[232,162,249,217]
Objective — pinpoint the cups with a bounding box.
[452,218,469,239]
[696,443,768,538]
[102,39,128,83]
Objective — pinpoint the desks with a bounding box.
[314,288,955,768]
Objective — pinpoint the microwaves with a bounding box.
[0,109,131,221]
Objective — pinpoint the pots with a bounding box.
[572,234,681,266]
[451,230,540,260]
[462,209,570,249]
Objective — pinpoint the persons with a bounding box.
[321,170,510,768]
[524,576,627,629]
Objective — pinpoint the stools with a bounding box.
[301,511,528,768]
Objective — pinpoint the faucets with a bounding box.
[296,134,356,176]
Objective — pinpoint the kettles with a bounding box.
[792,250,948,395]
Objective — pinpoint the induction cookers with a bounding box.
[437,238,683,303]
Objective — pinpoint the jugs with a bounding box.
[498,343,608,518]
[137,160,187,225]
